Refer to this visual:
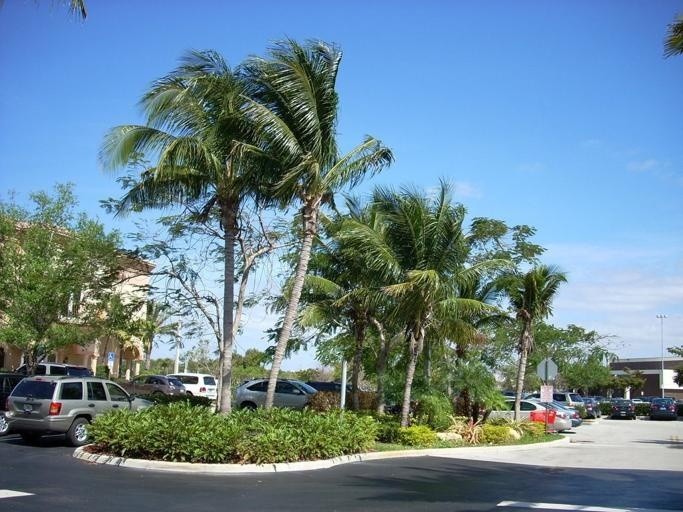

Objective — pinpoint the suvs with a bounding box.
[0,371,26,435]
[117,374,186,401]
[304,380,360,393]
[14,362,95,377]
[234,377,319,414]
[165,373,218,401]
[5,376,155,446]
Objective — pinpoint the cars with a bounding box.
[478,389,682,430]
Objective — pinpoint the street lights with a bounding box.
[174,298,187,375]
[654,313,668,398]
